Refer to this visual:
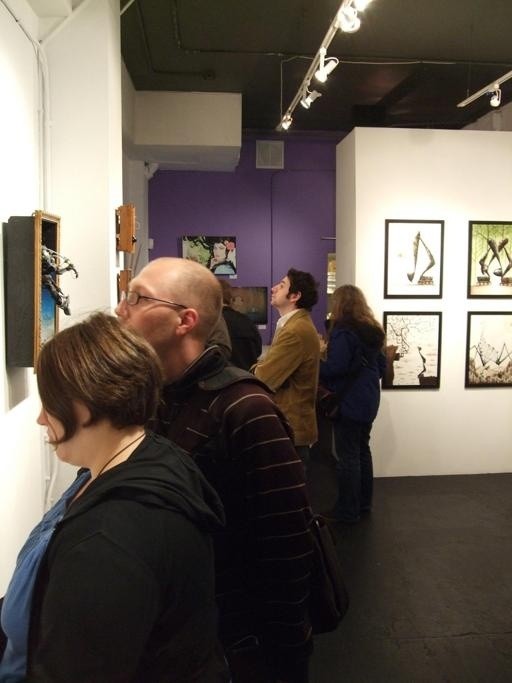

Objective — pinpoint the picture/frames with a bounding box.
[380,218,511,390]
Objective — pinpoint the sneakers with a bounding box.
[320,506,370,522]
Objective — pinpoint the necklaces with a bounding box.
[94,431,145,476]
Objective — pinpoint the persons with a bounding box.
[0,307,240,681]
[206,314,234,360]
[113,254,325,682]
[203,238,236,273]
[245,265,320,486]
[314,282,389,530]
[215,278,264,373]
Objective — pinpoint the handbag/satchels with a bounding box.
[309,512,350,634]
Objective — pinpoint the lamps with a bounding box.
[299,49,339,109]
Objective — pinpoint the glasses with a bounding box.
[120,290,187,310]
